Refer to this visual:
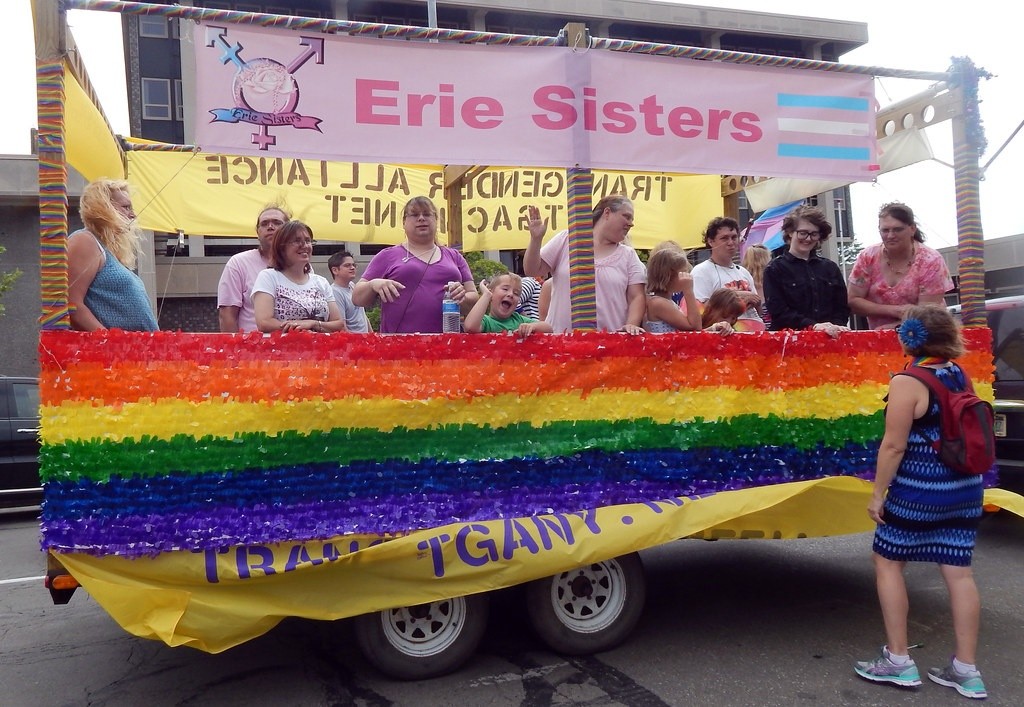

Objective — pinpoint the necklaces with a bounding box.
[881,247,915,273]
[402,240,437,262]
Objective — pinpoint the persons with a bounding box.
[327,251,373,333]
[68,181,161,331]
[740,244,771,330]
[352,196,480,333]
[763,205,850,339]
[525,195,646,336]
[848,202,955,330]
[690,217,764,324]
[855,306,988,698]
[217,206,314,332]
[463,250,553,336]
[646,240,702,331]
[249,220,344,333]
[701,288,746,336]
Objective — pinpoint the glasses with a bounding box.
[280,239,317,247]
[339,263,358,269]
[793,229,822,241]
[119,204,132,211]
[895,324,901,333]
[404,211,435,218]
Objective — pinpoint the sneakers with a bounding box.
[853,645,923,686]
[927,655,987,698]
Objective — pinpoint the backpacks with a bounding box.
[892,360,996,473]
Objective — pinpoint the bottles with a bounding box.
[442,281,460,333]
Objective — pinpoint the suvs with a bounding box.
[0,375,47,517]
[947,295,1023,496]
